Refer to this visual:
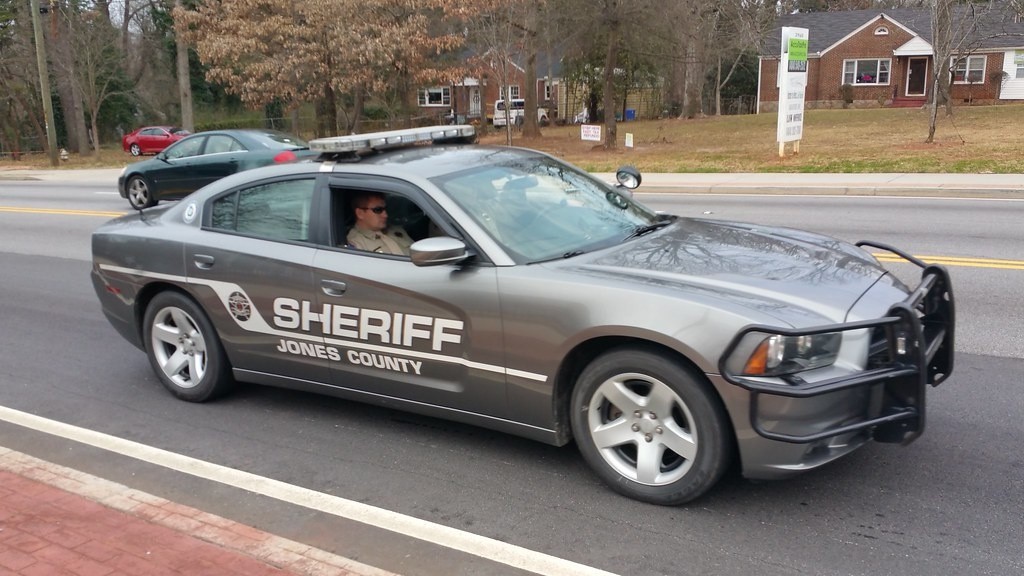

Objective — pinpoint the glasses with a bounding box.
[362,206,385,213]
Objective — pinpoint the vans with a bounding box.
[493,98,547,128]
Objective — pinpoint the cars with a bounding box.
[122,126,192,156]
[91,125,955,506]
[118,128,309,210]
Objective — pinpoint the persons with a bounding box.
[347,191,415,257]
[493,182,534,244]
